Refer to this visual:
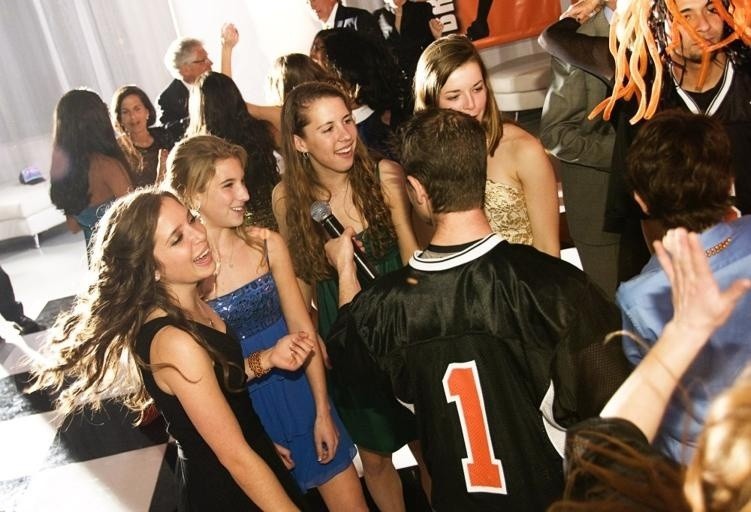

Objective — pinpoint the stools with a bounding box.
[1,176,67,250]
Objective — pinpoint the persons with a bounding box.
[0,266,42,333]
[51,1,751,511]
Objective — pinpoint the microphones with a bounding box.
[309,198,379,285]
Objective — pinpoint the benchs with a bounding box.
[478,35,551,125]
